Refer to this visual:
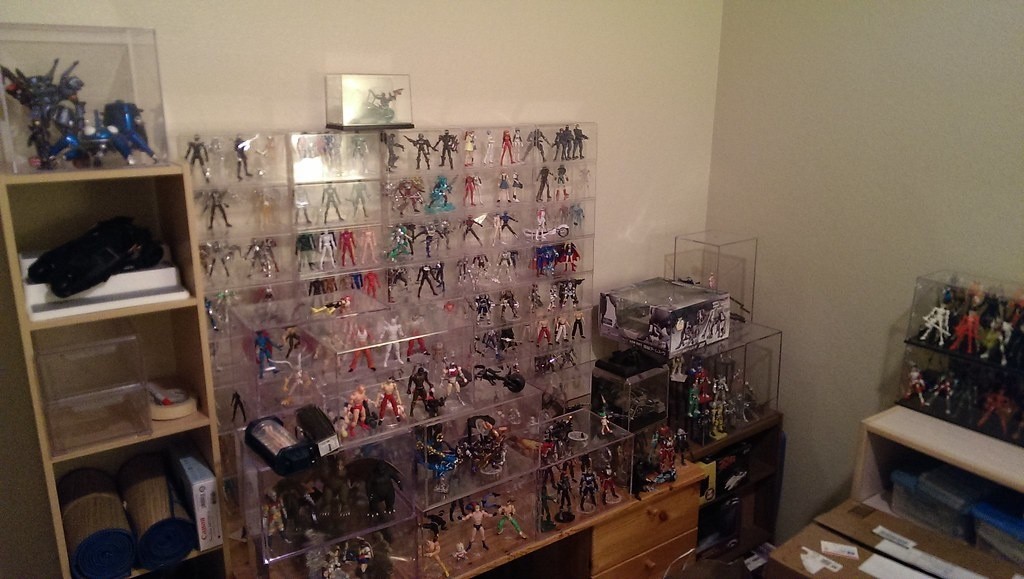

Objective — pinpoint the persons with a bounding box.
[0,58,159,170]
[185,87,773,578]
[902,272,1024,448]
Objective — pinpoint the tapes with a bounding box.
[151,383,197,420]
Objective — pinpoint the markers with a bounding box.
[148,385,172,405]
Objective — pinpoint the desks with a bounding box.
[768,406,1024,579]
[384,455,709,579]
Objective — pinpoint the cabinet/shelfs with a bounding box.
[896,271,1024,450]
[0,162,240,579]
[186,121,783,579]
